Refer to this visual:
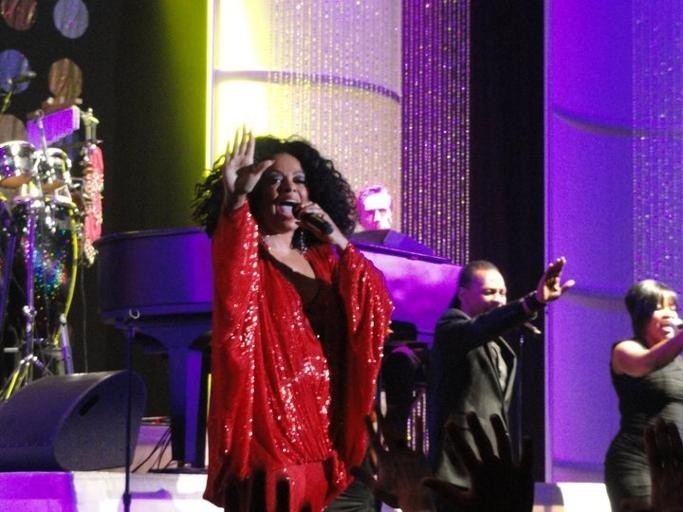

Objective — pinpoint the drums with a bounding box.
[0,140,72,192]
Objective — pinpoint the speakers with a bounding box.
[0,368,148,471]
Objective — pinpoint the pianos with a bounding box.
[91,226,466,468]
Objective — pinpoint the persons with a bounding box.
[348,401,536,511]
[420,255,576,512]
[190,125,396,511]
[604,276,683,511]
[621,416,683,512]
[354,185,431,450]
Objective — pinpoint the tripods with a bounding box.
[0,213,55,402]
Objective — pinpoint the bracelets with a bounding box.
[525,289,547,312]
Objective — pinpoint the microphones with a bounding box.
[496,303,543,339]
[292,203,332,234]
